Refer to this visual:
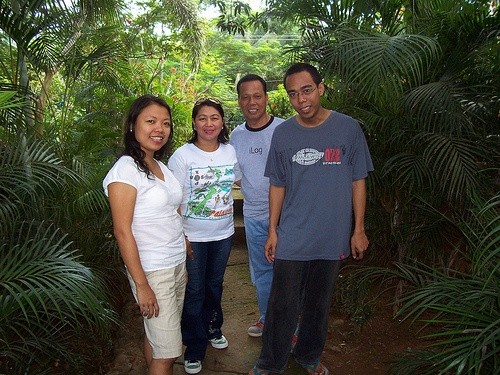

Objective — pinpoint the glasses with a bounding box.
[288,87,317,98]
[195,97,219,105]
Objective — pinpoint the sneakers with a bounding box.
[184,360,201,374]
[211,335,229,349]
[248,321,264,337]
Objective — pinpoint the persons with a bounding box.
[167,98,244,375]
[229,74,288,337]
[248,63,375,375]
[102,95,189,375]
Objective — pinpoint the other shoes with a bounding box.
[308,363,329,375]
[248,366,268,375]
